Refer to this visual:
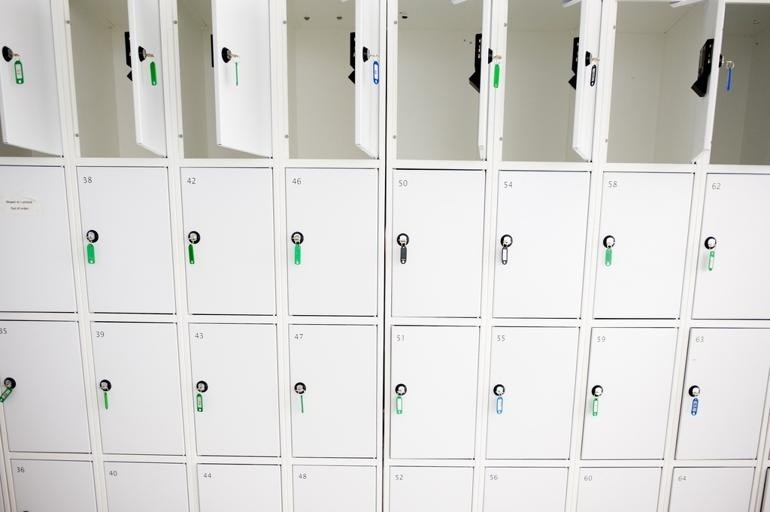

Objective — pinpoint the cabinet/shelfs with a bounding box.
[280,163,383,321]
[384,164,486,324]
[281,1,389,162]
[594,166,694,324]
[690,166,770,321]
[385,2,494,165]
[0,317,94,454]
[384,464,474,510]
[0,159,83,314]
[0,0,70,158]
[190,461,287,510]
[705,1,770,166]
[280,321,381,462]
[488,321,577,465]
[480,466,571,510]
[86,318,190,458]
[164,3,281,163]
[7,456,101,511]
[289,461,381,510]
[580,321,680,460]
[489,165,589,321]
[385,320,482,462]
[573,466,665,510]
[184,321,285,460]
[602,2,725,164]
[496,1,609,164]
[63,1,169,157]
[72,160,182,318]
[100,459,192,510]
[174,162,280,320]
[674,323,768,463]
[669,467,757,510]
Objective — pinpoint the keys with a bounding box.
[594,388,602,395]
[190,236,197,242]
[86,234,94,241]
[5,380,12,388]
[370,54,381,58]
[592,56,600,63]
[100,382,110,390]
[399,236,407,243]
[145,53,154,58]
[725,60,734,68]
[297,385,305,394]
[692,389,700,397]
[708,240,717,248]
[493,55,502,60]
[294,234,300,241]
[198,383,205,392]
[504,236,511,246]
[606,238,614,247]
[398,386,406,395]
[496,388,505,395]
[11,52,19,60]
[228,53,239,59]
